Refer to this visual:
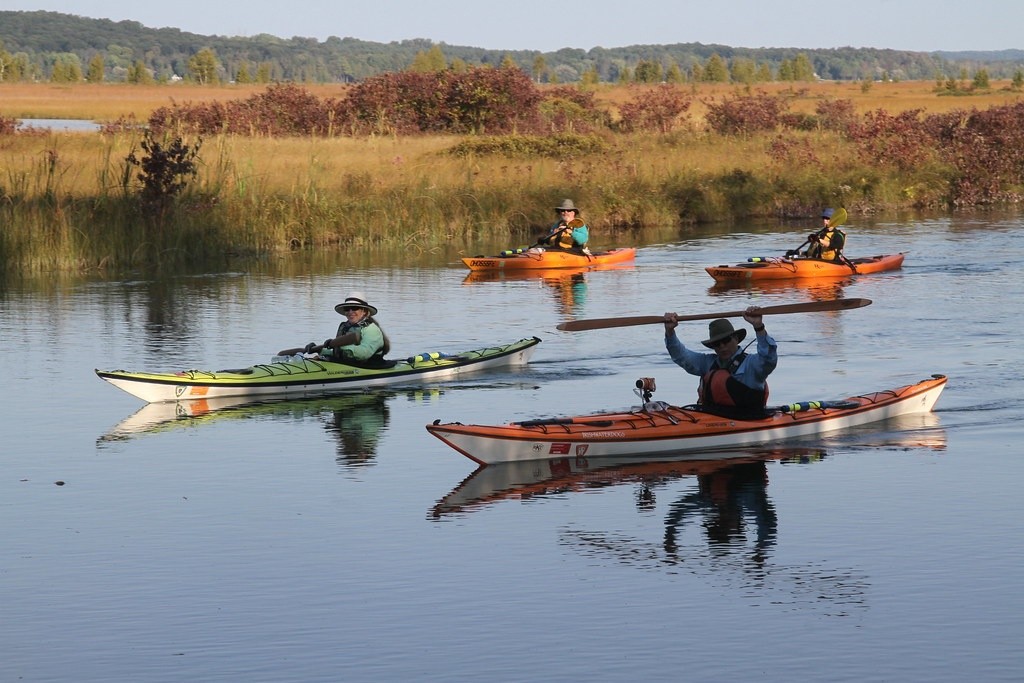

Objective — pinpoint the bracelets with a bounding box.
[754,323,765,332]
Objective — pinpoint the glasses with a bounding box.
[713,336,732,347]
[561,209,571,212]
[344,306,363,311]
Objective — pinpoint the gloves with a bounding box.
[786,249,799,258]
[538,237,544,245]
[808,234,820,243]
[324,339,331,348]
[303,343,315,354]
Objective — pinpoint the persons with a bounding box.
[537,199,589,256]
[786,208,844,262]
[664,306,778,421]
[301,291,390,368]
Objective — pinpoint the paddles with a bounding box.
[795,207,848,252]
[276,331,361,356]
[556,297,873,333]
[528,217,585,248]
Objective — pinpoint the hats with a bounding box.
[555,199,578,212]
[821,208,835,219]
[701,319,746,349]
[335,292,377,316]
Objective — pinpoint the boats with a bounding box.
[461,247,635,272]
[705,251,911,283]
[93,336,542,404]
[93,364,540,442]
[431,412,946,518]
[425,373,948,467]
